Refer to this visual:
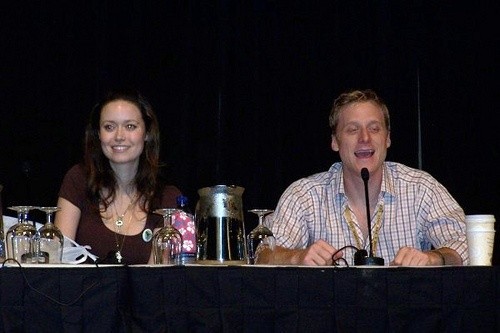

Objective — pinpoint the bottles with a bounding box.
[170,193,197,265]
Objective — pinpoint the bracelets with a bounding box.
[431,249,446,266]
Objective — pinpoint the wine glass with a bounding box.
[153,209,182,262]
[247,208,276,266]
[6,204,39,264]
[34,206,64,264]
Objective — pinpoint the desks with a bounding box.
[0,264,500,333]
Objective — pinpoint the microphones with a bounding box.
[361,167,384,266]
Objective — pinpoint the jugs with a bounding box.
[190,182,249,266]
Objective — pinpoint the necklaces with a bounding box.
[107,186,141,226]
[108,188,137,263]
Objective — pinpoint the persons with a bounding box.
[256,88,469,267]
[51,91,190,265]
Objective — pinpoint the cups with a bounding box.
[465,214,495,267]
